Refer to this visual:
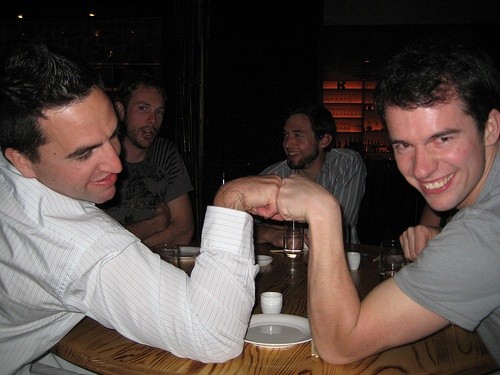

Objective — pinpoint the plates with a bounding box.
[169,246,201,262]
[243,313,312,348]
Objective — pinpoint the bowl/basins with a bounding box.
[256,255,273,266]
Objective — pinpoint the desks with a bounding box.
[49,241,500,375]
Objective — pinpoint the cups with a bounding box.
[346,251,361,271]
[260,292,282,314]
[151,243,179,268]
[283,220,303,259]
[378,239,406,280]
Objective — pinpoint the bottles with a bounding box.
[336,137,393,159]
[365,120,380,131]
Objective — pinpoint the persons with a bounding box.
[0,42,281,375]
[251,102,368,249]
[420,200,459,230]
[95,71,195,249]
[276,38,500,375]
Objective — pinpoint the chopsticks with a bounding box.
[270,249,283,253]
[310,340,319,358]
[373,256,381,262]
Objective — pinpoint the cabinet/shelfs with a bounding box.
[321,79,385,135]
